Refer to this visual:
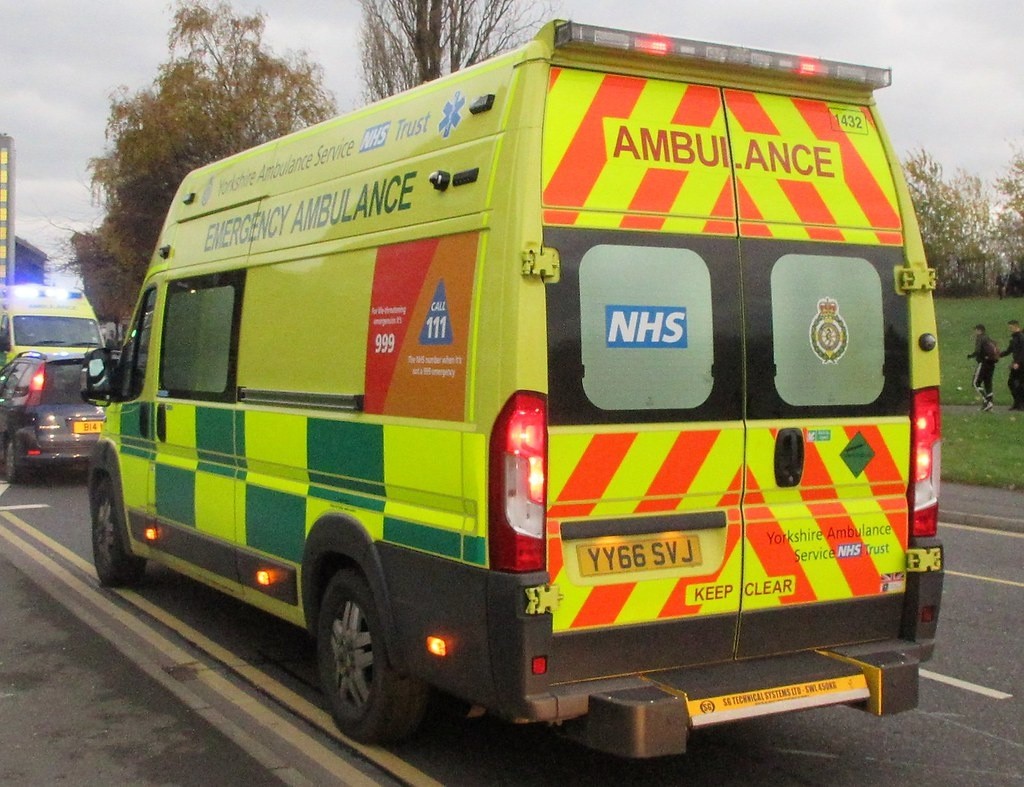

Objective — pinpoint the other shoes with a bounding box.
[1009,404,1022,412]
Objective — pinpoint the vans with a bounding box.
[1,283,114,372]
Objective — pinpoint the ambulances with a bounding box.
[77,17,941,761]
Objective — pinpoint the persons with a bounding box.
[999,320,1024,411]
[967,324,999,411]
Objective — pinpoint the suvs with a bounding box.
[1,349,119,485]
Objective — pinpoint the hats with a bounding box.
[973,324,984,330]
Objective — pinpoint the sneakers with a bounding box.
[981,401,992,410]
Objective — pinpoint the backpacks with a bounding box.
[981,338,999,365]
